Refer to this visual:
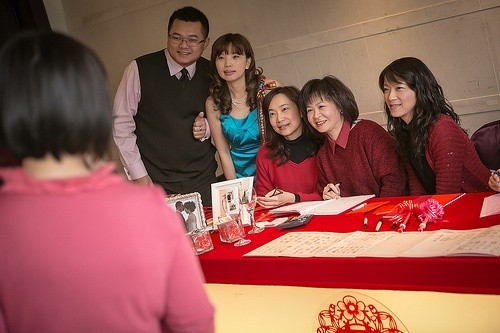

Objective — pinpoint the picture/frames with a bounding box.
[164,192,207,234]
[211,175,255,230]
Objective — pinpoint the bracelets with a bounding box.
[295,193,300,203]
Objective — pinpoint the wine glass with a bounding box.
[222,195,251,247]
[238,185,264,234]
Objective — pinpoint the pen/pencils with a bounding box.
[327,182,340,192]
[383,216,393,218]
[490,169,500,176]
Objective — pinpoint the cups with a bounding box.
[218,220,246,244]
[186,229,214,256]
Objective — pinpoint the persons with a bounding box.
[487,169,500,192]
[110,6,218,206]
[297,73,410,198]
[378,57,495,196]
[204,33,283,188]
[0,30,216,333]
[175,201,197,232]
[252,86,325,210]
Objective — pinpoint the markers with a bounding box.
[389,213,404,221]
[363,217,368,229]
[376,217,383,232]
[350,202,367,211]
[392,217,405,228]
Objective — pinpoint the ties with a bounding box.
[180,68,189,81]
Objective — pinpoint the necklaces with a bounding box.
[231,92,249,109]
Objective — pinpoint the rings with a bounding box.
[199,126,201,131]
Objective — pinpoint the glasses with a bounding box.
[169,34,203,47]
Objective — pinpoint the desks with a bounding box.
[195,192,500,333]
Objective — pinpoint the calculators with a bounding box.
[278,214,314,228]
